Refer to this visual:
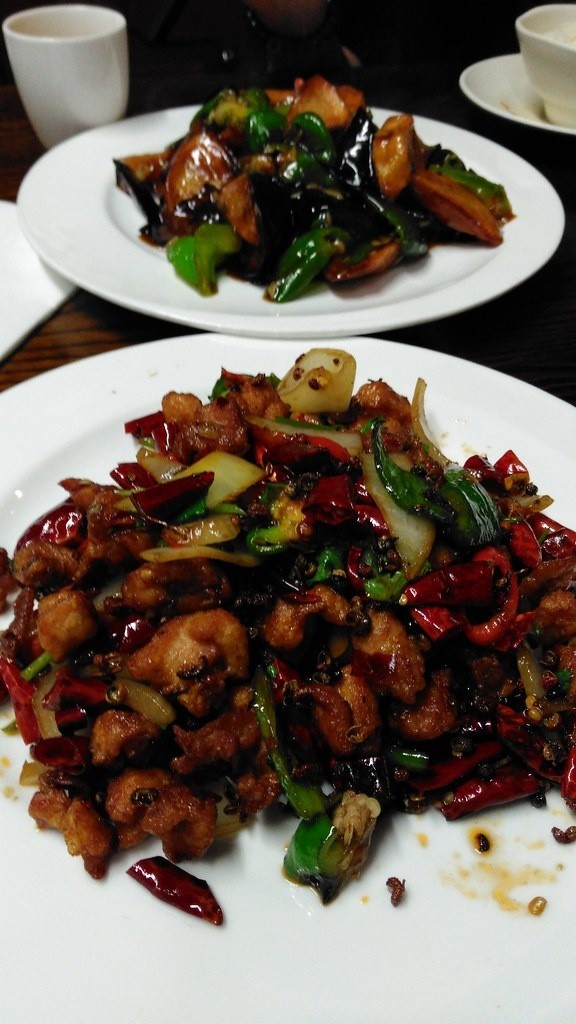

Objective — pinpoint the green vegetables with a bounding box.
[18,373,500,901]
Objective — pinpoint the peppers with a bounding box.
[163,83,512,303]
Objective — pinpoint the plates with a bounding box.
[460,53,576,136]
[0,333,576,1024]
[17,100,569,339]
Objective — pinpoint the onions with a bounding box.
[276,347,356,414]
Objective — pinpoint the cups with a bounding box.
[4,5,127,149]
[515,4,576,128]
